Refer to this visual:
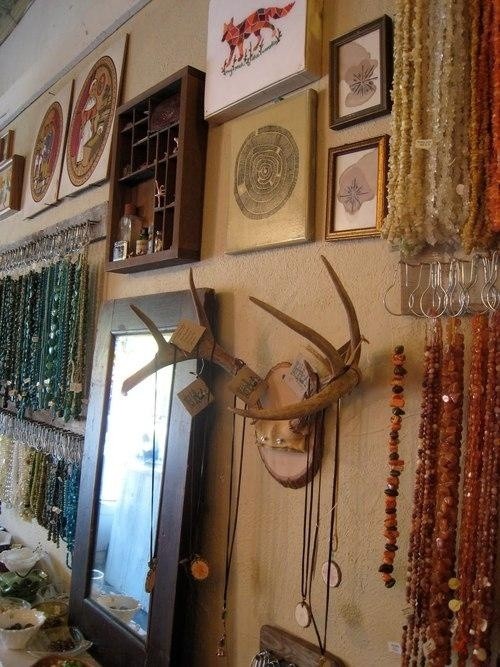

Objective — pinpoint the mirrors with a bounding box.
[65,288,216,667]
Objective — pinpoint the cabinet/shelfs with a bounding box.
[103,64,210,275]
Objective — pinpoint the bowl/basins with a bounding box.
[0,608,46,650]
[96,593,142,626]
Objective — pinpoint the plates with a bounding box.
[24,625,94,660]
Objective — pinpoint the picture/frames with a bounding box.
[0,129,25,220]
[325,13,393,243]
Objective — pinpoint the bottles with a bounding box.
[135,225,148,256]
[153,230,162,253]
[112,240,128,261]
[116,203,142,263]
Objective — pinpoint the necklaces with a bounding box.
[370,3,500,666]
[144,342,177,593]
[319,395,342,587]
[294,372,319,628]
[214,359,251,657]
[0,222,102,558]
[189,331,216,581]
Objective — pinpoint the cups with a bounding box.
[89,570,104,601]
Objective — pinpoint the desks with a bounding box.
[0,634,102,667]
